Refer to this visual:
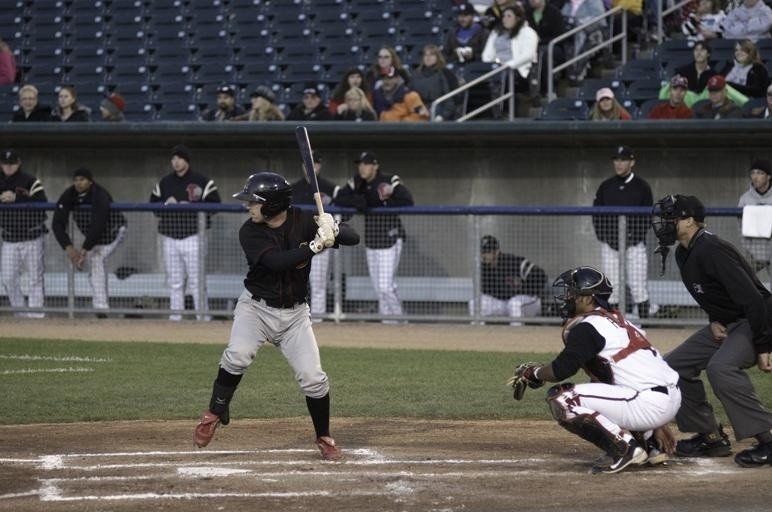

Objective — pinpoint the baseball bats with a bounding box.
[295,125,335,247]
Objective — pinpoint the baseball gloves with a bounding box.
[506,361,545,400]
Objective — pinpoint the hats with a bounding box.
[167,145,189,162]
[750,158,771,176]
[355,152,380,163]
[595,87,615,102]
[707,75,725,90]
[612,144,633,162]
[671,77,689,89]
[0,150,19,166]
[71,166,93,180]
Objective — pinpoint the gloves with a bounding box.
[309,213,340,254]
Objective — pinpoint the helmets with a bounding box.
[233,171,292,221]
[553,264,612,325]
[653,194,704,255]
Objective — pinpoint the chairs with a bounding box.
[1,0,771,122]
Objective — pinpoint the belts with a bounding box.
[252,294,306,309]
[651,385,668,394]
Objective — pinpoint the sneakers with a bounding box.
[592,441,649,473]
[192,411,219,444]
[644,439,670,465]
[676,433,733,457]
[736,446,771,468]
[315,435,345,462]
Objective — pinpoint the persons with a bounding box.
[739,161,772,261]
[194,173,360,461]
[335,151,414,323]
[0,151,49,318]
[51,170,127,320]
[506,267,682,473]
[592,147,654,329]
[291,155,340,322]
[470,236,545,324]
[651,194,772,469]
[149,145,222,323]
